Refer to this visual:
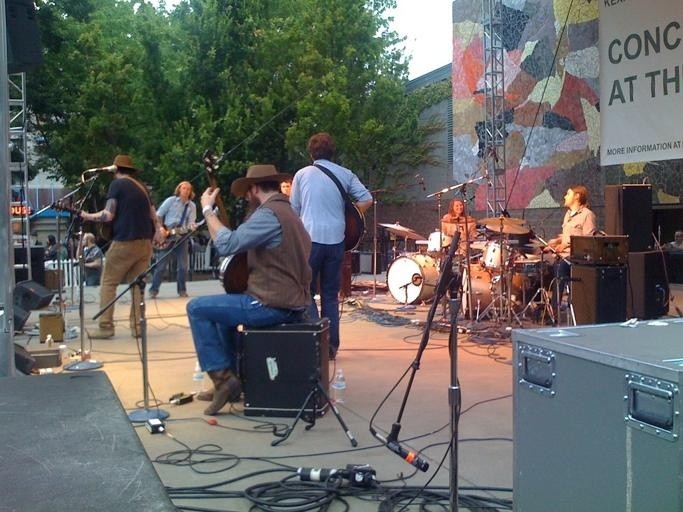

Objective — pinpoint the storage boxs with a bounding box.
[46,267,65,288]
[37,312,66,344]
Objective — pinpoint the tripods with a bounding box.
[271,368,358,448]
[436,229,570,328]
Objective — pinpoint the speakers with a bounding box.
[14,247,55,376]
[571,265,626,325]
[626,250,670,320]
[604,183,653,250]
[570,235,631,266]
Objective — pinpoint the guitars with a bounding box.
[47,201,114,254]
[345,198,363,254]
[204,151,249,294]
[151,222,198,251]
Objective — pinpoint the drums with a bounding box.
[386,251,440,304]
[461,264,506,319]
[511,254,539,294]
[482,240,512,267]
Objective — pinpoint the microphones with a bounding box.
[370,189,385,194]
[419,182,427,191]
[486,169,492,187]
[198,206,219,226]
[88,164,118,174]
[370,427,430,473]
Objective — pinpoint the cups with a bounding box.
[59,345,69,362]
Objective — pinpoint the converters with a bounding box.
[145,418,166,434]
[169,392,193,405]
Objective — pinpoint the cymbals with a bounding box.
[442,217,474,223]
[381,221,426,240]
[525,243,548,247]
[485,224,529,235]
[479,217,525,224]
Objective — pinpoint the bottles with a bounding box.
[193,360,205,392]
[45,335,55,350]
[331,369,346,403]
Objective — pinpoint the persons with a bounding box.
[279,177,291,196]
[43,235,56,260]
[148,181,196,299]
[184,166,311,418]
[547,184,595,326]
[75,233,105,286]
[288,133,372,360]
[79,155,156,339]
[441,199,471,253]
[59,240,69,260]
[670,231,681,249]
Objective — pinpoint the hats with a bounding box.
[112,154,143,177]
[230,164,291,200]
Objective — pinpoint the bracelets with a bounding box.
[201,205,213,216]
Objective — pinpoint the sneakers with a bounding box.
[132,325,143,338]
[203,372,240,416]
[88,324,114,339]
[149,292,189,299]
[199,388,241,401]
